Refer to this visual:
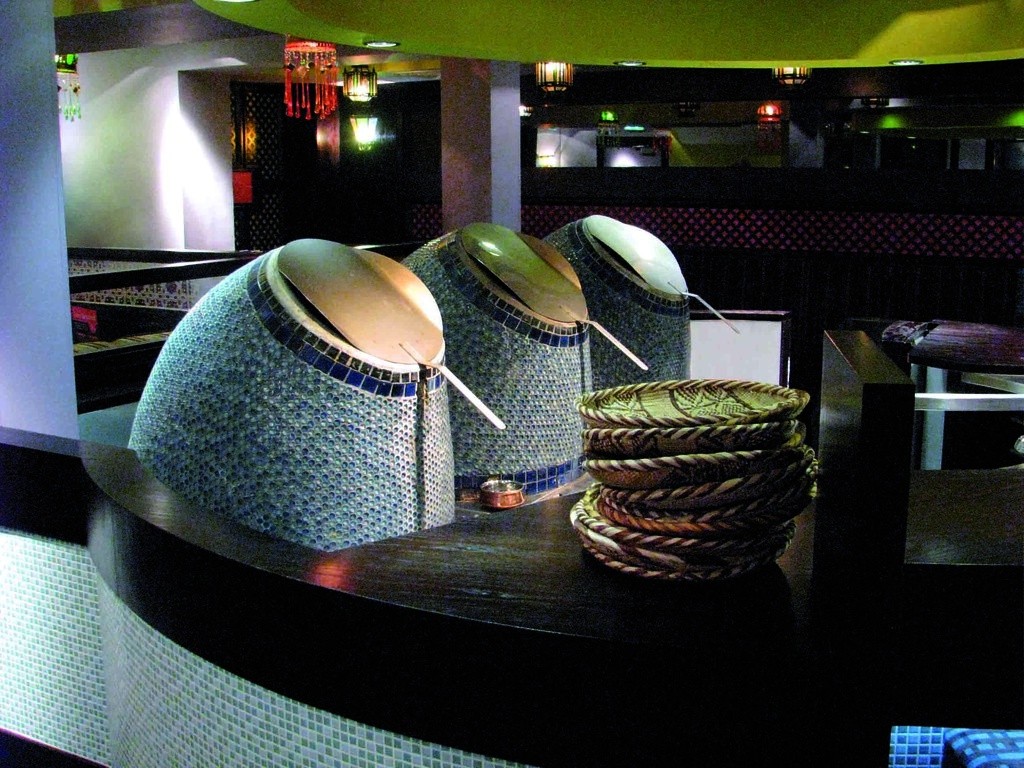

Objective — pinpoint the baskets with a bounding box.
[568,377,820,581]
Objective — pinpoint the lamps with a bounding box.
[757,100,782,128]
[598,111,620,149]
[56,53,82,123]
[283,36,340,121]
[349,113,378,151]
[772,66,811,85]
[534,61,574,93]
[342,65,379,111]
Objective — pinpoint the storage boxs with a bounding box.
[125,214,819,589]
